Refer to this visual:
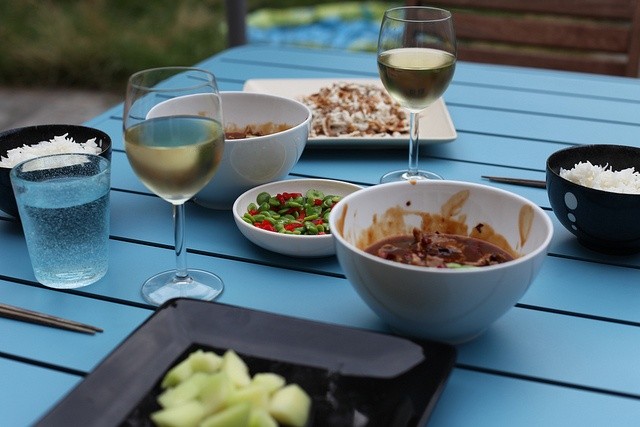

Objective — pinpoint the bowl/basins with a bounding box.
[0,124,112,222]
[148,94,311,209]
[546,145,637,255]
[329,179,555,352]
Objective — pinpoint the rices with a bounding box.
[1,132,105,171]
[560,161,637,195]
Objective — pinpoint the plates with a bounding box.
[34,297,456,427]
[247,76,457,141]
[231,180,363,254]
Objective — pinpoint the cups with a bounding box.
[11,152,111,291]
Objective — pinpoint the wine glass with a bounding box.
[378,6,468,184]
[124,67,223,303]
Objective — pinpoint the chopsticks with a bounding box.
[484,175,548,190]
[0,304,104,337]
